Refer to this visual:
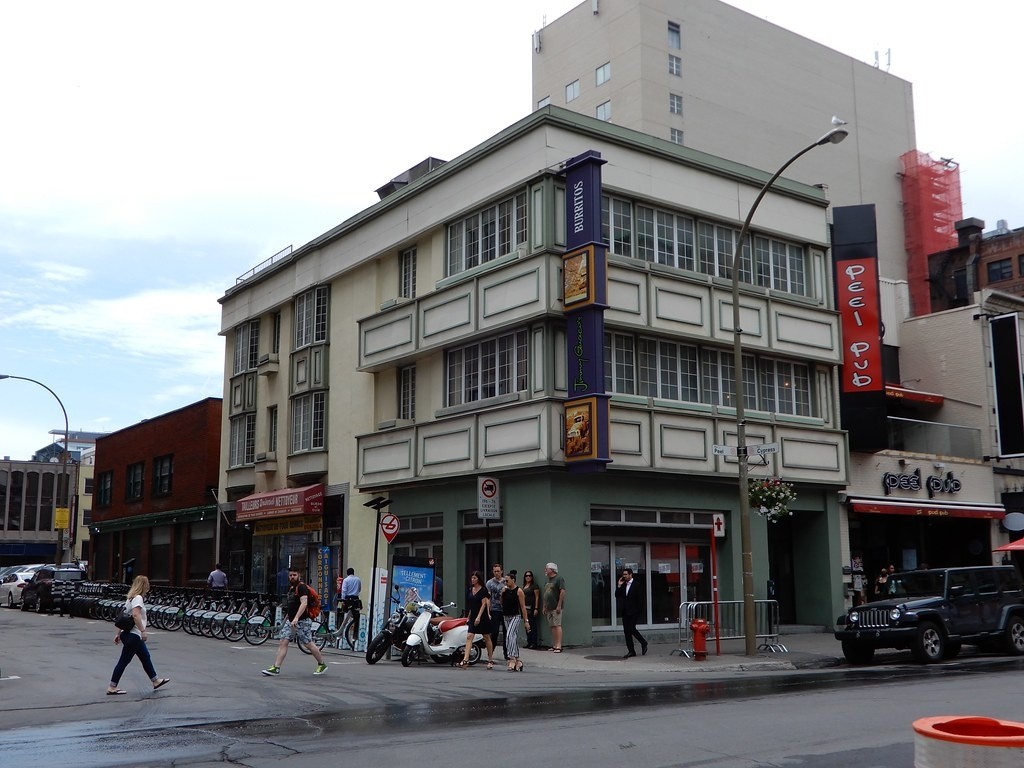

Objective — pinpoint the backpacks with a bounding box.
[295,582,320,619]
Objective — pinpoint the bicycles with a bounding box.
[69,580,290,646]
[296,594,370,656]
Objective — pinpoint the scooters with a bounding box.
[366,586,456,666]
[401,586,487,668]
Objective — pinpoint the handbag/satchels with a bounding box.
[115,597,136,633]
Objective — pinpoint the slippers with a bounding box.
[107,689,127,695]
[154,678,170,689]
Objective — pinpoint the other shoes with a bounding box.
[528,644,537,649]
[523,644,530,648]
[487,661,494,670]
[456,659,470,668]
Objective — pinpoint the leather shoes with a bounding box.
[623,651,636,659]
[641,641,648,655]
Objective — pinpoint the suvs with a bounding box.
[833,566,1024,664]
[20,564,89,613]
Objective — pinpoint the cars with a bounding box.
[0,562,75,610]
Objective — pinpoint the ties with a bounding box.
[626,582,630,595]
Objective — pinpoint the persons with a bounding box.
[500,569,531,672]
[106,575,170,695]
[342,568,361,643]
[262,565,328,676]
[456,571,494,671]
[888,565,900,598]
[207,563,228,591]
[522,571,540,650]
[486,564,511,664]
[72,557,86,571]
[615,568,648,658]
[542,562,566,653]
[875,569,889,601]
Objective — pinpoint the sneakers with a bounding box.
[313,662,329,675]
[262,664,280,676]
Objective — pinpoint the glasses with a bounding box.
[504,580,508,583]
[525,575,532,577]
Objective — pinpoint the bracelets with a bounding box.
[525,619,529,623]
[534,608,538,610]
[141,629,146,632]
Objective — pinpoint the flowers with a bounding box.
[748,474,797,523]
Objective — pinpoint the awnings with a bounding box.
[235,482,324,522]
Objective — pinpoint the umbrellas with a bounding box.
[991,536,1024,551]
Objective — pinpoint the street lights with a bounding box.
[0,373,69,567]
[731,126,852,657]
[362,495,393,651]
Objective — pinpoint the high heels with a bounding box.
[507,662,516,671]
[515,661,523,672]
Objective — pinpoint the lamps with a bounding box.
[201,512,205,520]
[173,516,178,523]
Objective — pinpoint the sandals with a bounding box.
[554,649,562,653]
[548,646,556,651]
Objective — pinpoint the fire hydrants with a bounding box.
[688,618,710,661]
